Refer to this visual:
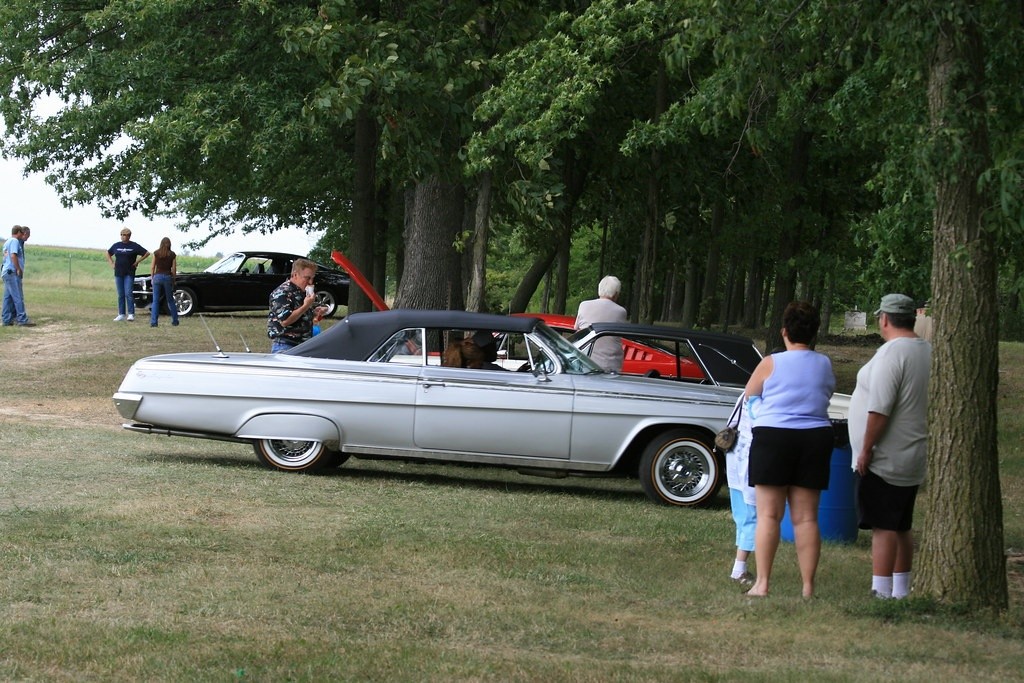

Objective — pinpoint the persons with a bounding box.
[443,331,531,372]
[573,275,627,373]
[745,300,836,600]
[1,225,38,327]
[105,228,150,322]
[150,238,180,327]
[848,295,931,617]
[726,392,758,587]
[267,259,328,354]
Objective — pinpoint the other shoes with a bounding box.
[127,315,134,320]
[114,315,125,320]
[18,323,36,327]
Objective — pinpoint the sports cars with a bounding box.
[111,251,853,507]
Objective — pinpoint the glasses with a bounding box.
[121,234,129,236]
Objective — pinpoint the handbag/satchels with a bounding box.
[713,427,735,452]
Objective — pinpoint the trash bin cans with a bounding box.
[780,416,858,542]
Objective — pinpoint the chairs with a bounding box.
[266,262,275,274]
[253,263,265,274]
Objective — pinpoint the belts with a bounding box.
[273,339,297,347]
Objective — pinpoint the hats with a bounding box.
[873,294,916,315]
[471,331,500,347]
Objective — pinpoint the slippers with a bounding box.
[729,571,756,585]
